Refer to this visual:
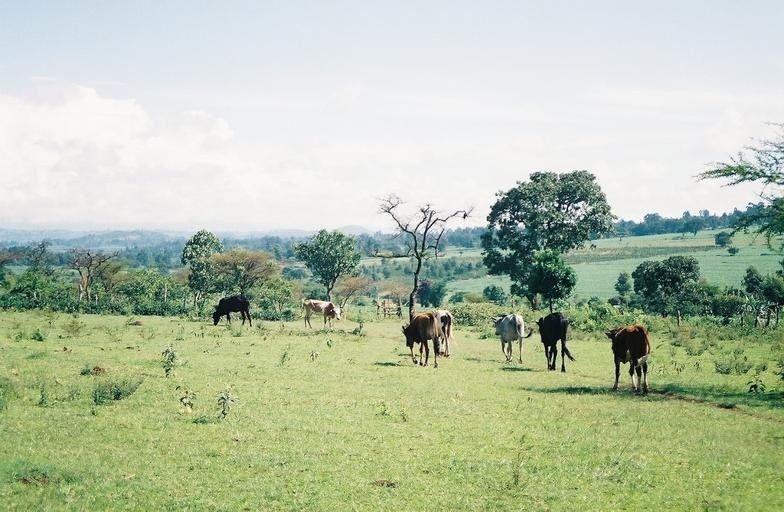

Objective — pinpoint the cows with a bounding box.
[211,294,252,327]
[605,324,651,394]
[535,312,576,372]
[303,299,338,329]
[401,309,453,368]
[493,313,534,364]
